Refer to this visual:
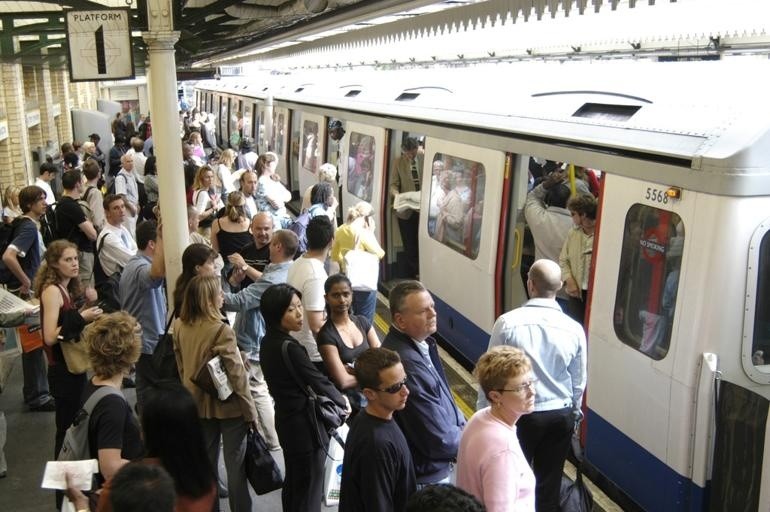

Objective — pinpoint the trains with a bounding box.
[192,55,770,511]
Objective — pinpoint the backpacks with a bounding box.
[0,216,32,286]
[38,200,79,249]
[79,172,128,226]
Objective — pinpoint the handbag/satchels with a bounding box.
[57,322,92,376]
[13,288,45,355]
[281,340,346,460]
[54,385,137,494]
[244,422,285,497]
[321,420,351,507]
[559,409,593,512]
[188,323,236,404]
[89,230,129,311]
[145,300,180,390]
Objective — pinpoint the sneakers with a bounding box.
[29,397,55,412]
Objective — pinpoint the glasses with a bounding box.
[493,376,540,395]
[368,375,410,395]
